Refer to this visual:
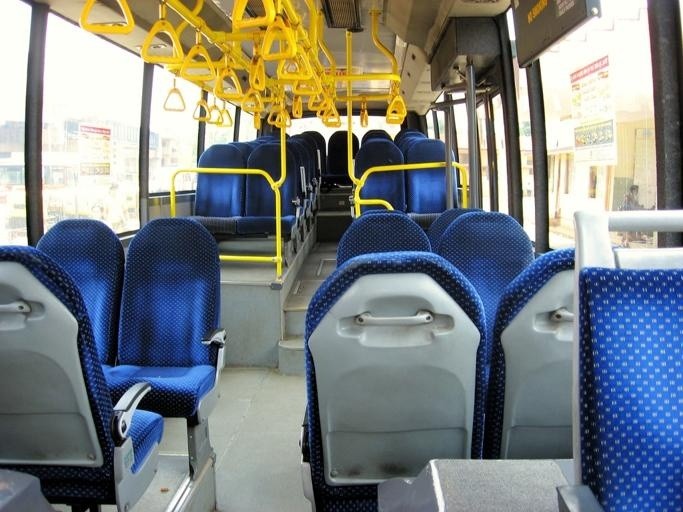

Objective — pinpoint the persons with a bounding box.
[619,185,647,243]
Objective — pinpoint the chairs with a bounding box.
[301,205,576,512]
[557,207,682,511]
[1,242,166,512]
[35,217,225,482]
[355,129,461,235]
[185,131,326,267]
